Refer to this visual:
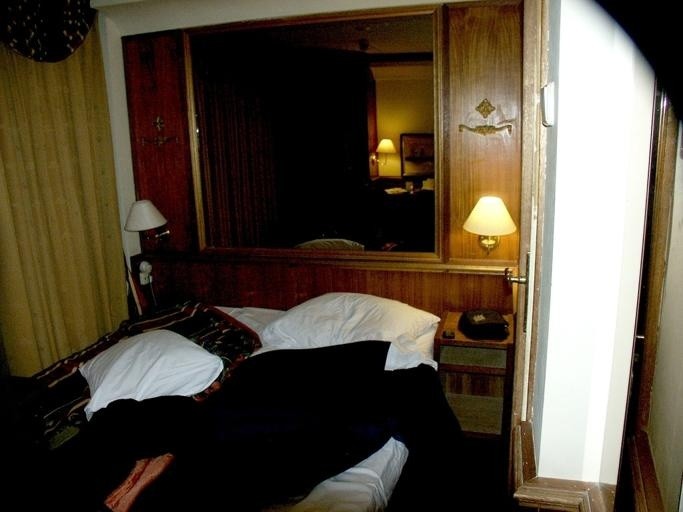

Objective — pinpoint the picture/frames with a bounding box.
[400,133,434,177]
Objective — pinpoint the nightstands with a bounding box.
[434,310,514,445]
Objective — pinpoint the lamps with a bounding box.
[371,138,396,166]
[124,200,170,240]
[463,196,517,255]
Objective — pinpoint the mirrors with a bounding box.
[182,3,446,265]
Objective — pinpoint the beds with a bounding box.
[0,305,438,512]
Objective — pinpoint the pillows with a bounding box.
[262,291,441,348]
[79,329,224,421]
